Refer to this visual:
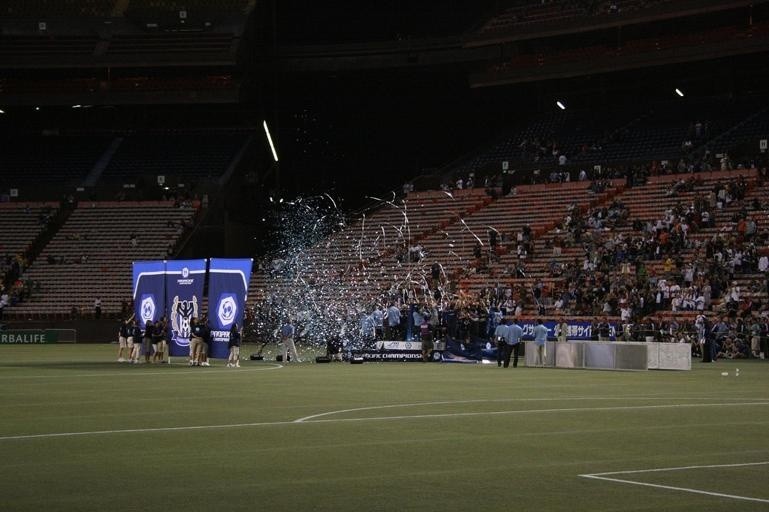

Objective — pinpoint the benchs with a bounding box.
[1,164,768,344]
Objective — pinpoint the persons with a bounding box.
[24,203,33,213]
[81,253,88,265]
[131,232,137,248]
[94,295,102,318]
[47,255,56,265]
[0,252,40,312]
[73,232,80,240]
[327,117,767,367]
[281,318,301,364]
[116,314,243,370]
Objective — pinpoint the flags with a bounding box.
[165,257,208,359]
[209,255,255,360]
[131,259,166,356]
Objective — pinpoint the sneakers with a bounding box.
[116,357,244,369]
